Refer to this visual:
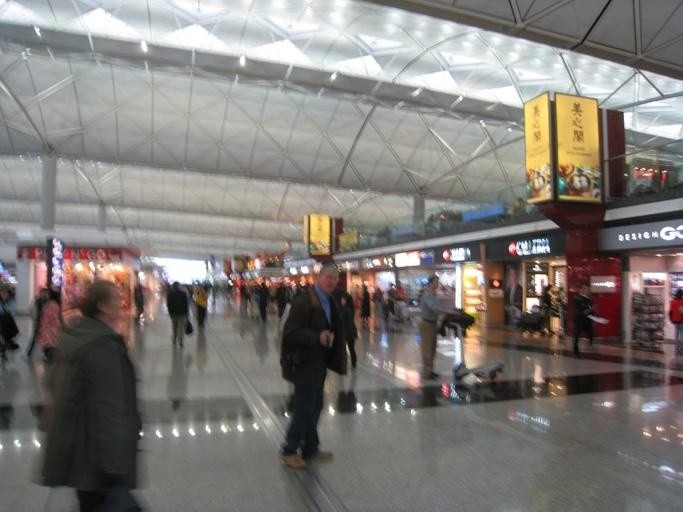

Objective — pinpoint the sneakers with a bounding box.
[306,451,333,464]
[279,452,306,469]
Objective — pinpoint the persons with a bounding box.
[255,325,269,361]
[240,280,303,323]
[133,283,145,324]
[28,288,64,364]
[506,277,523,316]
[572,283,595,355]
[194,282,209,327]
[668,288,683,355]
[167,345,188,408]
[166,281,189,349]
[28,279,144,511]
[276,262,346,467]
[334,278,359,368]
[541,285,552,334]
[0,283,19,360]
[195,327,208,369]
[361,323,397,372]
[418,277,439,380]
[361,283,404,326]
